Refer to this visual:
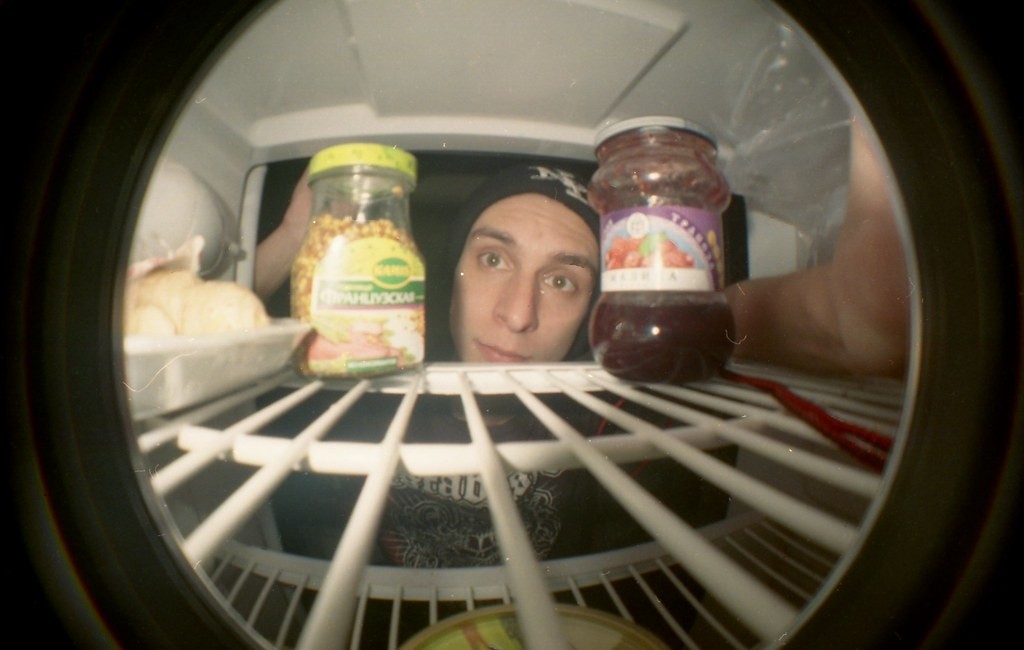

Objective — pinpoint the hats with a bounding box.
[434,163,603,298]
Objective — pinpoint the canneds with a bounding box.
[289,145,425,378]
[590,117,736,381]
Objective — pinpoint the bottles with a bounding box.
[289,142,426,381]
[586,114,736,384]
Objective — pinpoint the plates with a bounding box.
[123,306,313,423]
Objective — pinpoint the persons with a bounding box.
[254,106,913,650]
